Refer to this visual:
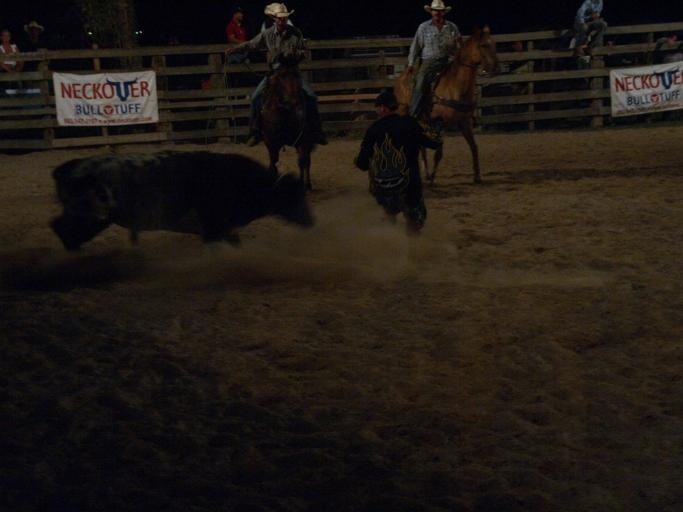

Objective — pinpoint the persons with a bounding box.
[1,1,683,147]
[354,92,426,237]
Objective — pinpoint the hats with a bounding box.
[424,0,451,13]
[25,21,44,34]
[265,3,294,18]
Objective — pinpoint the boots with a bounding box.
[305,109,328,145]
[247,115,259,146]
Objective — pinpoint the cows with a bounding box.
[50,150,314,252]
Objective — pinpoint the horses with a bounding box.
[392,24,497,187]
[262,52,313,192]
